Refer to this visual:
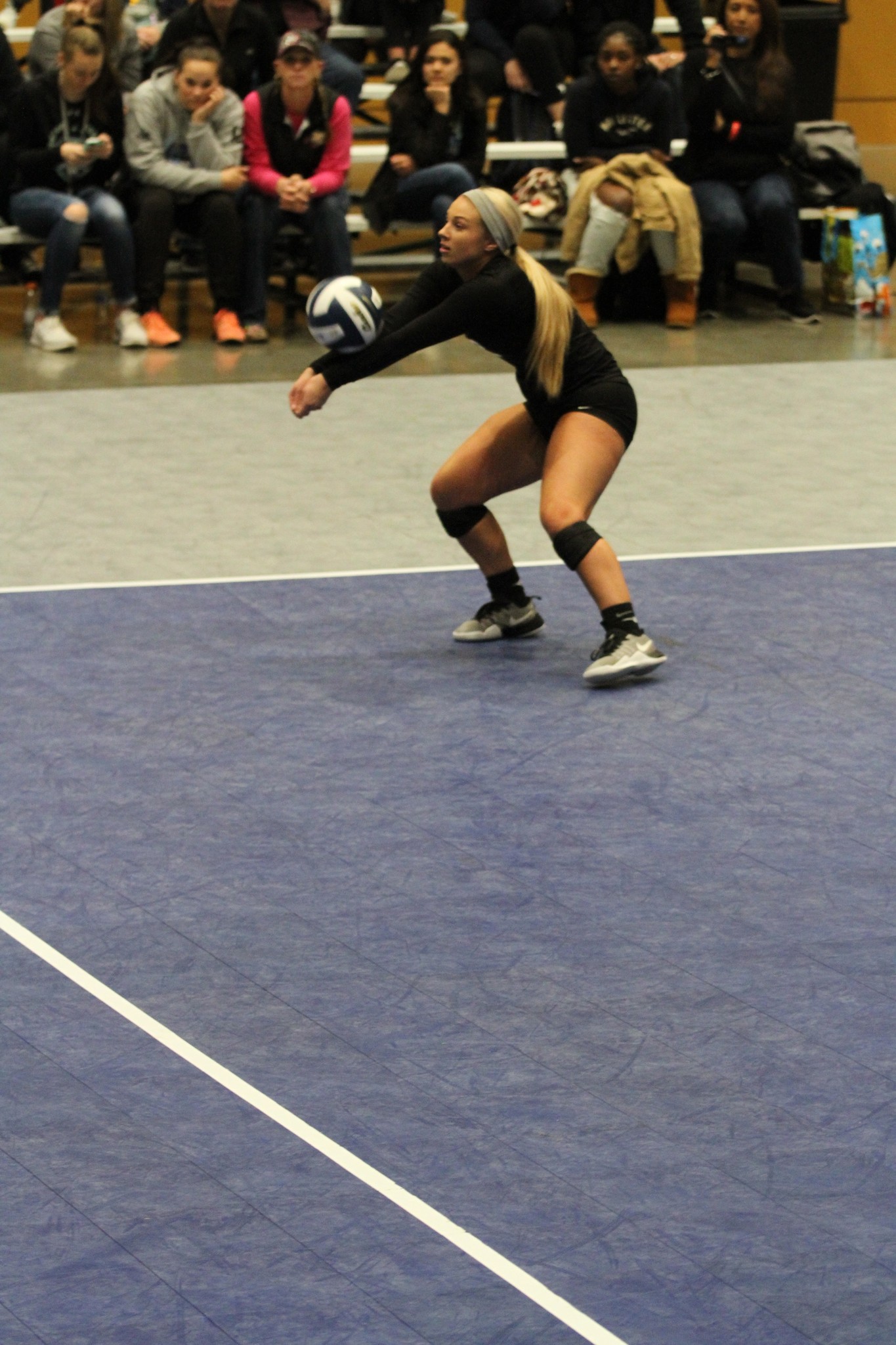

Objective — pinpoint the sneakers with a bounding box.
[452,593,545,642]
[581,623,667,684]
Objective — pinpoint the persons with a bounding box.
[2,0,849,352]
[288,184,667,685]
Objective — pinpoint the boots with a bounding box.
[564,268,604,328]
[660,269,698,328]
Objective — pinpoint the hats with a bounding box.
[278,29,325,63]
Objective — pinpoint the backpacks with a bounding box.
[794,120,862,196]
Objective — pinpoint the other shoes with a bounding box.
[244,322,269,346]
[113,310,148,347]
[212,308,246,344]
[28,314,79,354]
[140,308,181,349]
[775,288,824,324]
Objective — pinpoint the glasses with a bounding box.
[279,53,315,69]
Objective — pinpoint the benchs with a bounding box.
[0,0,856,323]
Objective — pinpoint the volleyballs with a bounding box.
[306,275,386,353]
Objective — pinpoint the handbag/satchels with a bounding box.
[820,206,890,320]
